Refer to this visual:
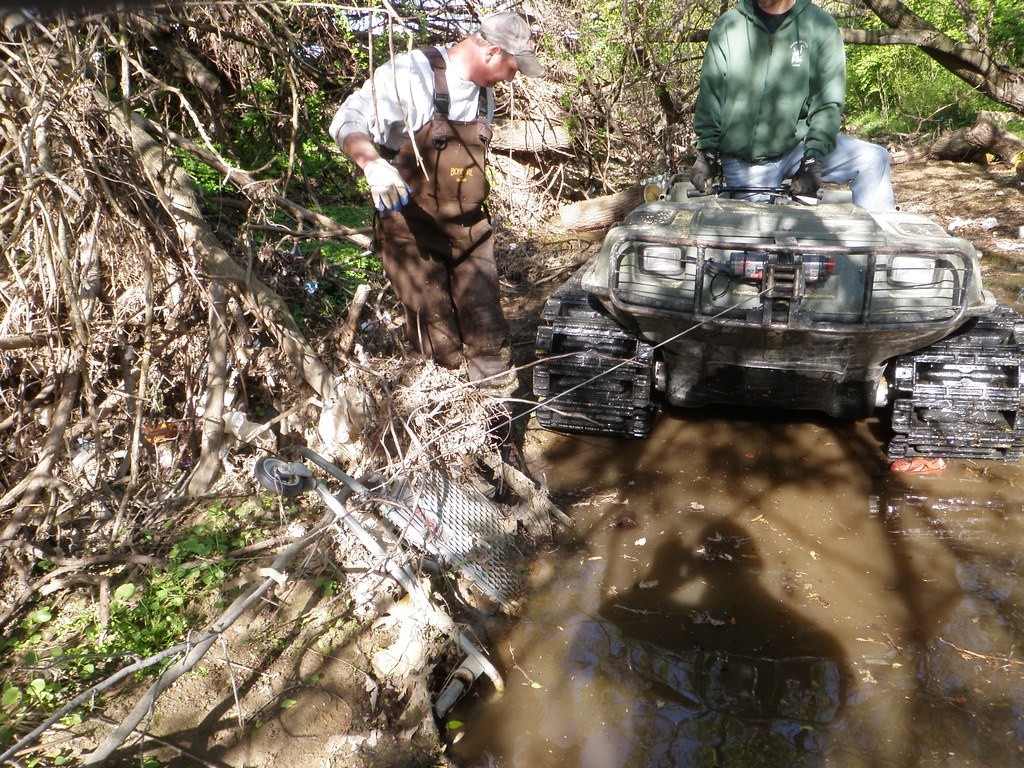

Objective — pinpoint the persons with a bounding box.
[690,0,894,212]
[328,12,541,496]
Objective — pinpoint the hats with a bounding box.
[480,13,546,78]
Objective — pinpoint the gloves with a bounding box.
[363,158,412,212]
[791,157,822,195]
[690,147,722,194]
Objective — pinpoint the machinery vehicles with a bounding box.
[534,161,1024,466]
